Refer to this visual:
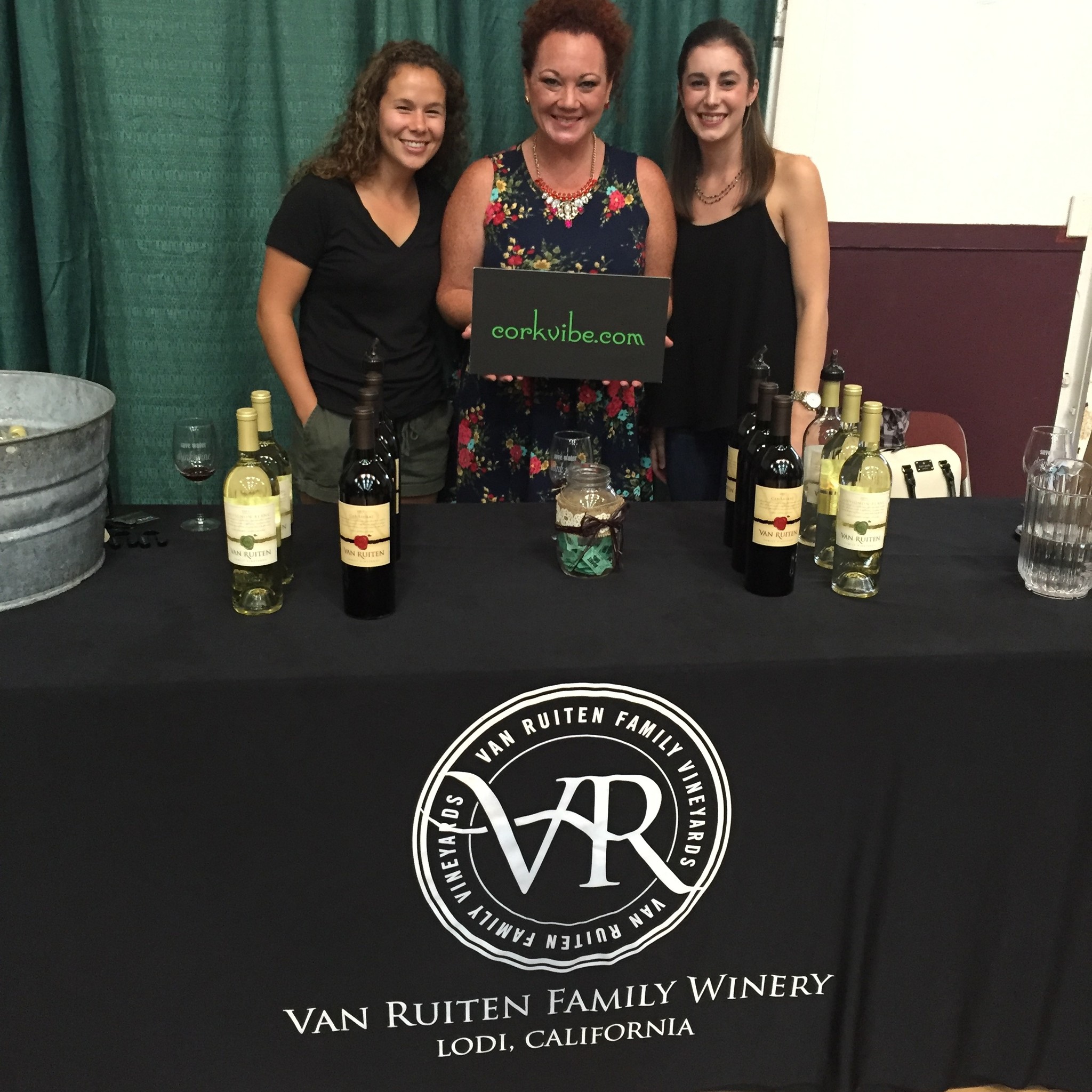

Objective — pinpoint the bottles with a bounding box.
[722,361,804,597]
[801,366,893,598]
[553,463,624,579]
[335,372,404,620]
[250,389,298,585]
[219,407,283,616]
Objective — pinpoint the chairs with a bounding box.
[880,407,972,497]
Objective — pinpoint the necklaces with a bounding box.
[694,168,743,204]
[532,131,598,229]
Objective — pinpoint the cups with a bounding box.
[548,431,593,488]
[1016,459,1092,600]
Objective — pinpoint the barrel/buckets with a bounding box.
[0,369,118,614]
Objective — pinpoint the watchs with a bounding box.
[790,391,822,411]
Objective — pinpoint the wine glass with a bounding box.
[171,417,222,533]
[1014,425,1074,535]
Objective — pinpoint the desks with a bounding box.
[2,506,1092,1092]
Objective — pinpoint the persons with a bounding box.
[650,17,830,501]
[255,38,468,505]
[436,0,678,503]
